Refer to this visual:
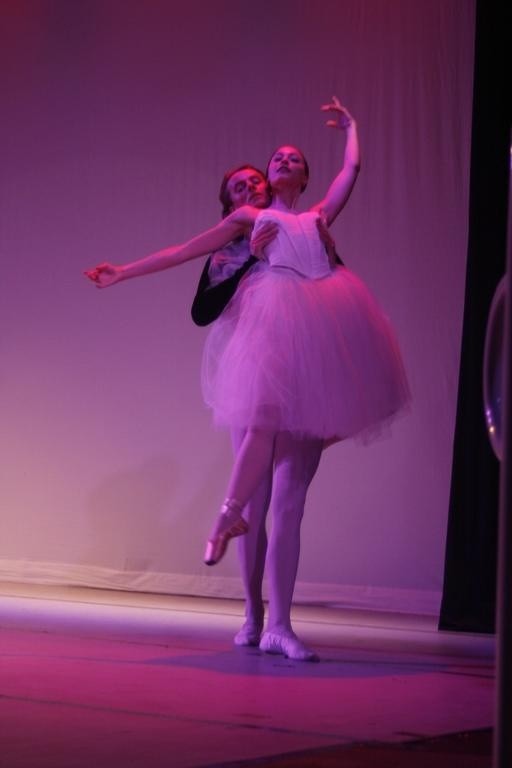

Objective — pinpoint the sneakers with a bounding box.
[261,632,316,660]
[204,505,250,566]
[234,621,263,646]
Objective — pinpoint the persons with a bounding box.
[81,90,418,566]
[187,162,348,665]
